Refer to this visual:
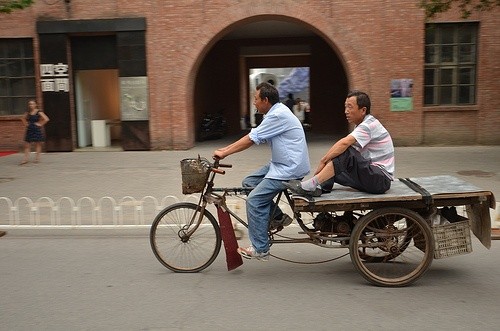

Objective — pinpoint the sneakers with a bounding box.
[238,245,269,262]
[269,213,293,229]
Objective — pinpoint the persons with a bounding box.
[282,90,394,199]
[20,99,49,164]
[214,82,310,261]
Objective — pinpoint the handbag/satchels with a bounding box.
[181,154,210,195]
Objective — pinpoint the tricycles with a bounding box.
[150,155,491,287]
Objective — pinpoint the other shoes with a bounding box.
[19,160,29,165]
[32,159,41,166]
[281,179,321,196]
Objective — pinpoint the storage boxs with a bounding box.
[412,211,473,260]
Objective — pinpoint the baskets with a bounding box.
[182,158,211,194]
